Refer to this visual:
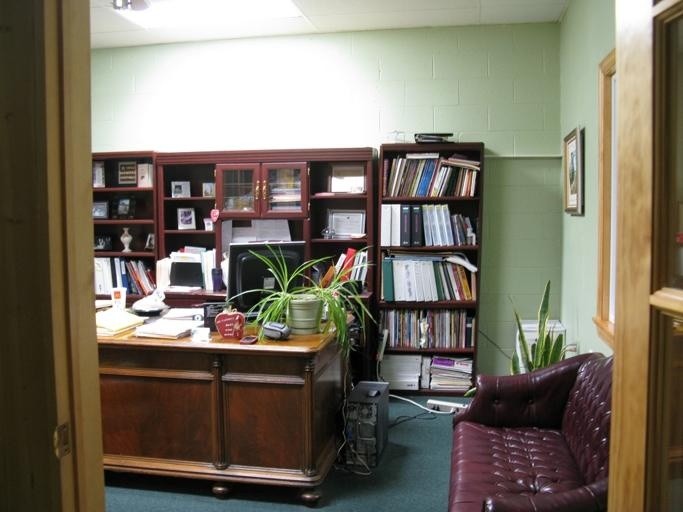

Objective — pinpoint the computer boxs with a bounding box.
[345,380,389,466]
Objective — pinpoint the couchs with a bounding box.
[447,354,612,511]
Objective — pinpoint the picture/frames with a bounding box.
[563,126,583,216]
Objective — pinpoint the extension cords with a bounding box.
[427,399,469,415]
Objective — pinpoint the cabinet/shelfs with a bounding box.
[517,321,566,375]
[375,143,483,395]
[91,150,159,303]
[157,147,378,381]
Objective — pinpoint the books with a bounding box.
[93,257,157,295]
[319,248,369,288]
[96,306,204,340]
[168,262,203,290]
[378,152,481,391]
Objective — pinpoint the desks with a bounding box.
[96,304,354,509]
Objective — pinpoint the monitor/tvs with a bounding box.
[225,241,307,324]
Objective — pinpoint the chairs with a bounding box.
[237,251,300,319]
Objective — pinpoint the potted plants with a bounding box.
[229,246,377,357]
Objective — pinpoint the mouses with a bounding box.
[192,313,204,322]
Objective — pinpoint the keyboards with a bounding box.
[206,305,259,320]
